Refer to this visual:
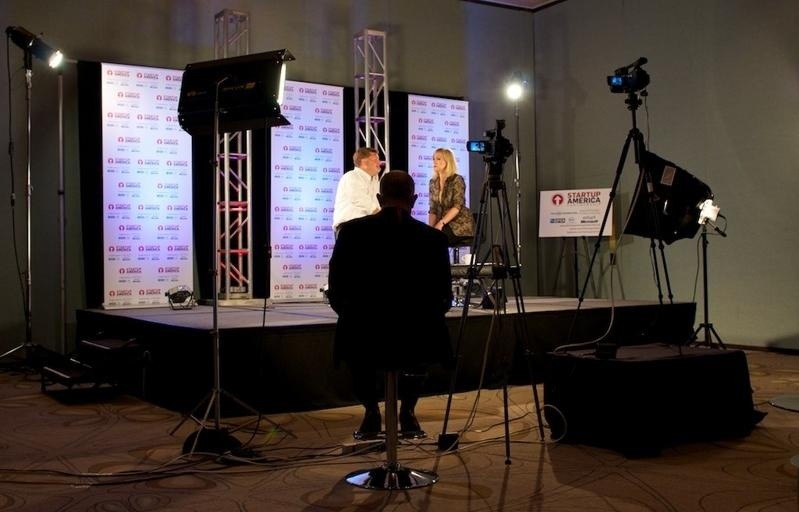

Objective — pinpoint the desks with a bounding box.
[543,340,771,462]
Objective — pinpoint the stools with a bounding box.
[346,358,441,491]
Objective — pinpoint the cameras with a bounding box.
[607,72,649,92]
[467,138,514,155]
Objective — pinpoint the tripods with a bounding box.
[0,47,66,384]
[562,92,682,358]
[170,78,298,460]
[682,228,728,351]
[438,153,545,463]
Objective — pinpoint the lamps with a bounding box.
[502,63,524,268]
[168,40,304,452]
[622,156,733,350]
[0,22,65,372]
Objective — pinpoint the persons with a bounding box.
[330,148,384,240]
[327,168,455,443]
[428,146,479,247]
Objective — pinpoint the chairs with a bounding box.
[445,211,487,308]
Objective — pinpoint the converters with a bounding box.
[439,432,458,450]
[341,439,385,454]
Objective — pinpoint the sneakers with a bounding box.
[350,411,383,438]
[398,408,424,436]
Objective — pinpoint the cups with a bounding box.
[462,254,477,265]
